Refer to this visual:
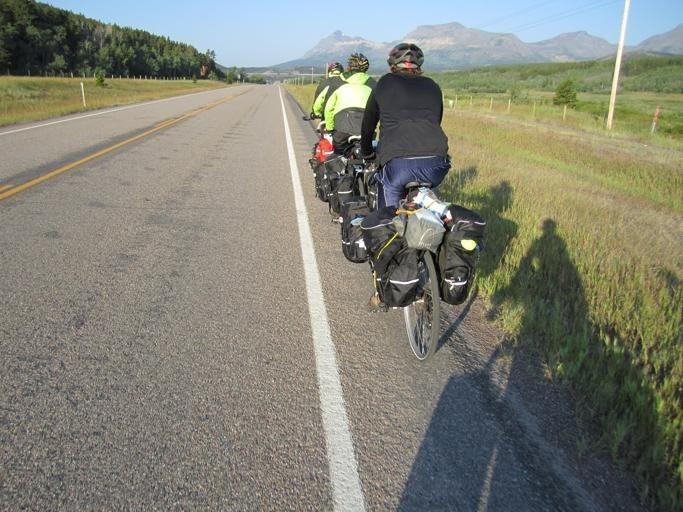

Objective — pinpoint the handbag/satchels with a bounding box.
[308,139,489,309]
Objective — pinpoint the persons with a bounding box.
[358,42,452,311]
[310,61,345,121]
[320,51,380,224]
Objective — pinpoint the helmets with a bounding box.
[387,44,424,68]
[327,62,342,76]
[346,54,371,70]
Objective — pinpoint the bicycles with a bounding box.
[302,111,444,363]
